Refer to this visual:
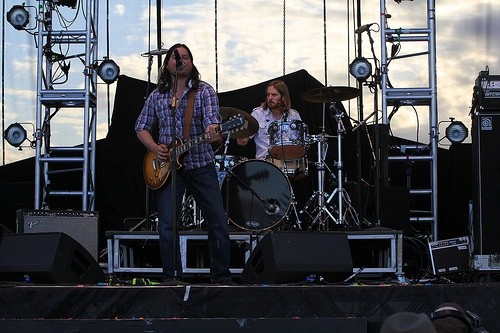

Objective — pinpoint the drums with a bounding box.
[268,155,306,174]
[221,159,293,233]
[266,121,306,160]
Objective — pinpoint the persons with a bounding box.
[135,43,235,286]
[237,80,308,177]
[380,303,477,333]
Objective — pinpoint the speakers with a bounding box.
[472,112,500,256]
[0,231,106,286]
[16,209,99,265]
[240,232,353,286]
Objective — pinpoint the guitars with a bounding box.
[142,114,245,190]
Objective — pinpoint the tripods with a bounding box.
[291,92,362,231]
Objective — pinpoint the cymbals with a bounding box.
[218,106,259,139]
[302,85,361,102]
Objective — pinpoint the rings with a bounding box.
[208,136,210,139]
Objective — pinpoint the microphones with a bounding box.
[352,112,375,133]
[173,49,185,70]
[141,49,168,56]
[354,23,373,34]
[267,204,277,213]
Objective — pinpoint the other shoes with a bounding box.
[220,277,239,286]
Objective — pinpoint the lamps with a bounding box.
[238,231,353,284]
[6,2,30,31]
[445,117,469,145]
[348,56,373,82]
[3,122,33,151]
[0,232,106,286]
[91,56,121,85]
